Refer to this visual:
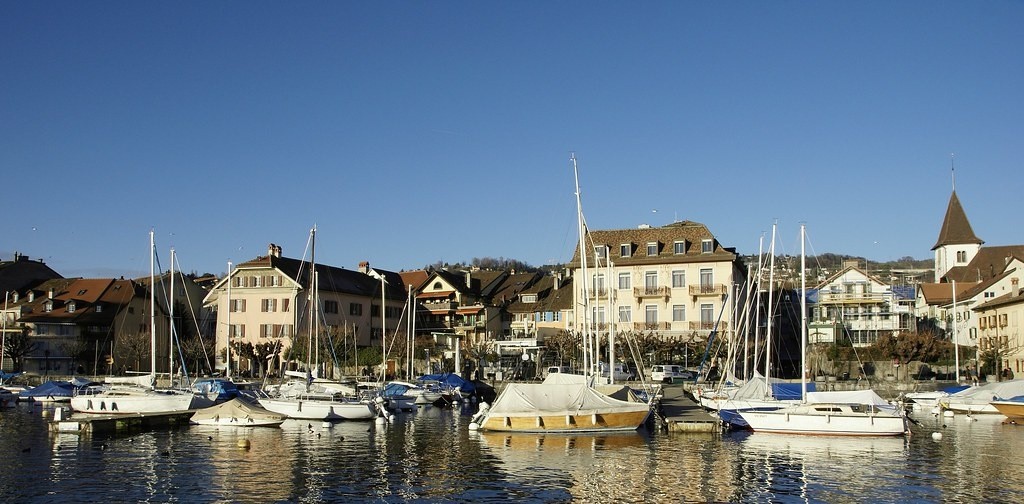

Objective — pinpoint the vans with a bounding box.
[547,365,571,373]
[651,364,693,384]
[603,362,637,381]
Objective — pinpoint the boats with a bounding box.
[189,397,288,427]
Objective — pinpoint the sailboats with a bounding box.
[681,223,906,436]
[0,229,241,415]
[256,228,477,421]
[904,279,1024,427]
[469,151,663,433]
